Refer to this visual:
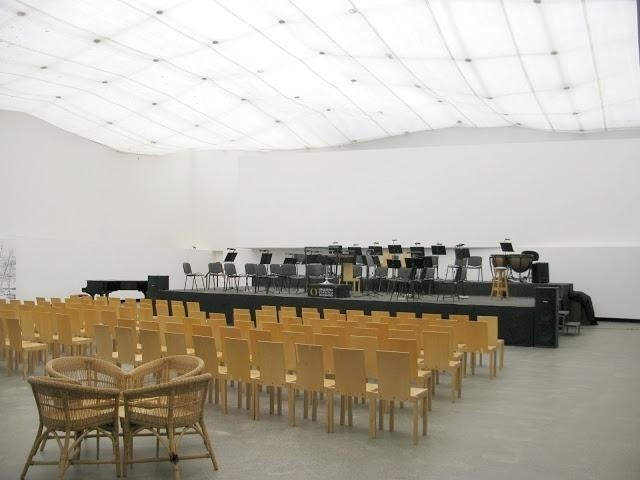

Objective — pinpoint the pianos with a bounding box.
[87,280,147,297]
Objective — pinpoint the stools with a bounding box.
[490,267,512,303]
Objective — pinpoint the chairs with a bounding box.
[20,355,219,479]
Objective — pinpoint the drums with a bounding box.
[492,255,508,267]
[508,254,533,272]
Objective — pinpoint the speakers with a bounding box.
[532,263,549,283]
[148,276,169,299]
[534,286,559,349]
